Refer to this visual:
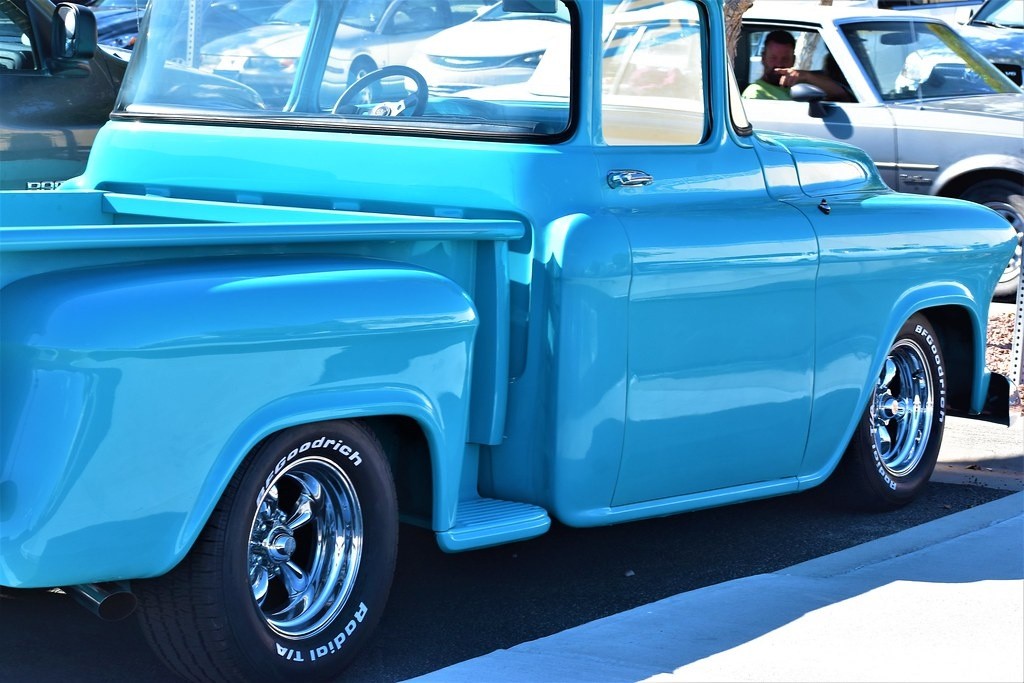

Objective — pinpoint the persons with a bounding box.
[742,30,854,102]
[823,29,869,80]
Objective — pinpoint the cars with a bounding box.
[0,0,1022,683]
[71,0,1023,310]
